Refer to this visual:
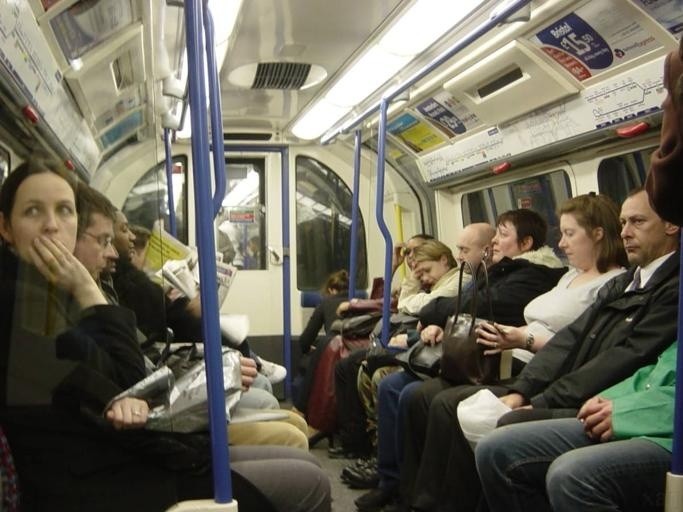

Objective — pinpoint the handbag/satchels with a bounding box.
[330,310,443,376]
[439,314,502,386]
[104,342,243,438]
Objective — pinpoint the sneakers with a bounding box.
[257,355,287,385]
[327,446,408,511]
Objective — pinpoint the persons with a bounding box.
[1,158,331,512]
[642,33,683,229]
[296,183,682,512]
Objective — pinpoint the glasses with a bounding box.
[81,227,114,249]
